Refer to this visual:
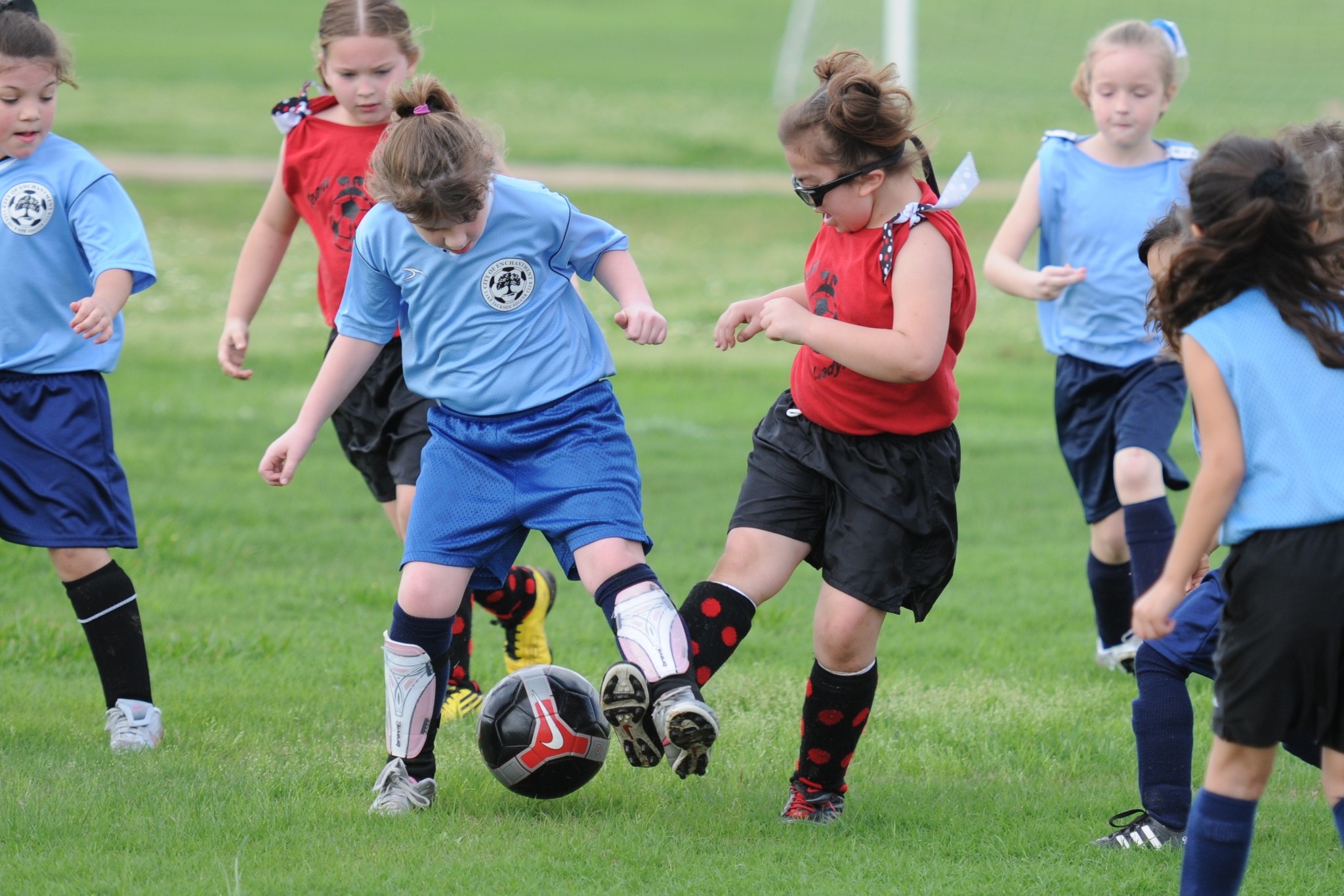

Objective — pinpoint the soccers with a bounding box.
[477,666,610,800]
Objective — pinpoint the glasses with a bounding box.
[790,169,862,208]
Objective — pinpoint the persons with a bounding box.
[221,0,555,719]
[0,0,164,747]
[599,48,980,823]
[1094,118,1344,895]
[257,75,718,817]
[985,19,1199,672]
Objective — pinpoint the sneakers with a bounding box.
[598,660,664,770]
[438,683,484,729]
[663,687,721,780]
[780,783,847,821]
[1096,633,1144,679]
[502,564,557,675]
[369,768,436,818]
[1095,816,1192,850]
[106,698,163,754]
